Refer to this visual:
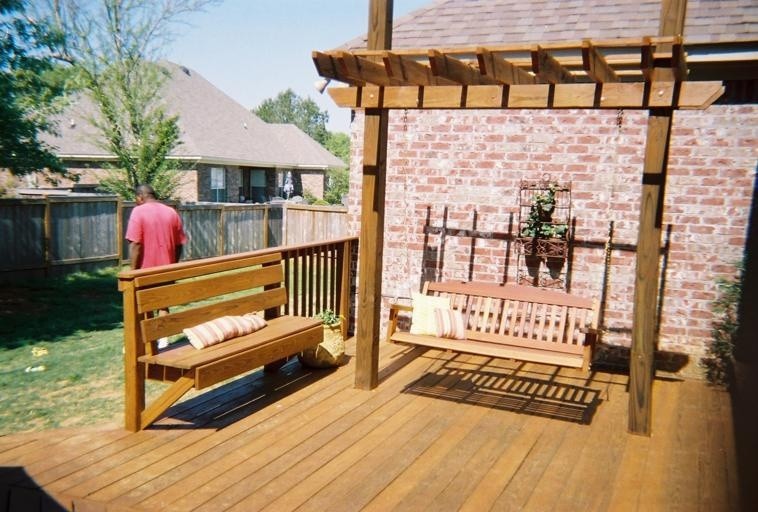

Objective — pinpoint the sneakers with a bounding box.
[157,337,170,349]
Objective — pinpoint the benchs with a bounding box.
[136,252,327,429]
[385,278,604,376]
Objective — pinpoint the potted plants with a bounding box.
[520,186,567,280]
[297,307,346,368]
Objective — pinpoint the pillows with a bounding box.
[179,311,268,351]
[409,290,466,341]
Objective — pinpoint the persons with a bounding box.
[122,183,188,359]
